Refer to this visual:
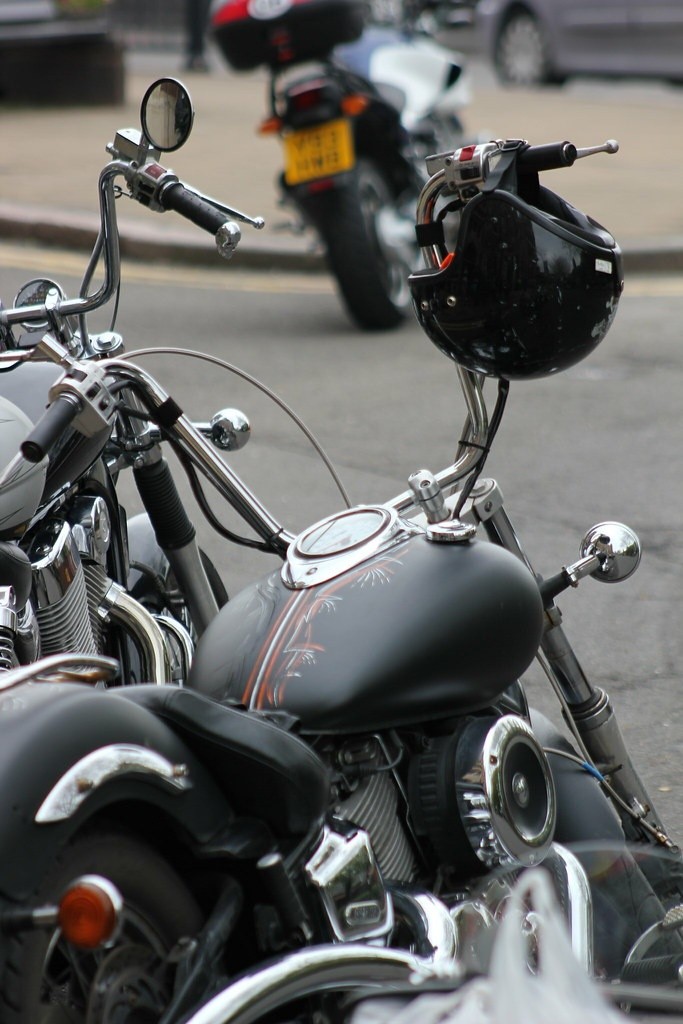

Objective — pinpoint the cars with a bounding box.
[420,1,683,89]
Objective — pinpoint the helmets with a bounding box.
[407,188,622,381]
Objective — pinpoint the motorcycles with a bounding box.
[1,74,683,1024]
[201,0,475,330]
